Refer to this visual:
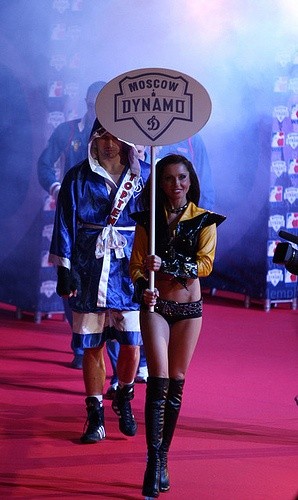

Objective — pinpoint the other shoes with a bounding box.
[136,366,149,382]
[106,381,119,397]
[72,354,84,368]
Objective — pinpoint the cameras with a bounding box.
[272,242,298,277]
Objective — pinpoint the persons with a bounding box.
[36,80,227,498]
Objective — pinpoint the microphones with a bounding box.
[278,231,298,244]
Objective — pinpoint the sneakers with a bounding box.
[80,396,106,443]
[112,380,139,436]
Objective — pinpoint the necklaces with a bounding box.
[168,202,188,213]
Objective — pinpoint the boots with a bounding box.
[141,376,185,497]
